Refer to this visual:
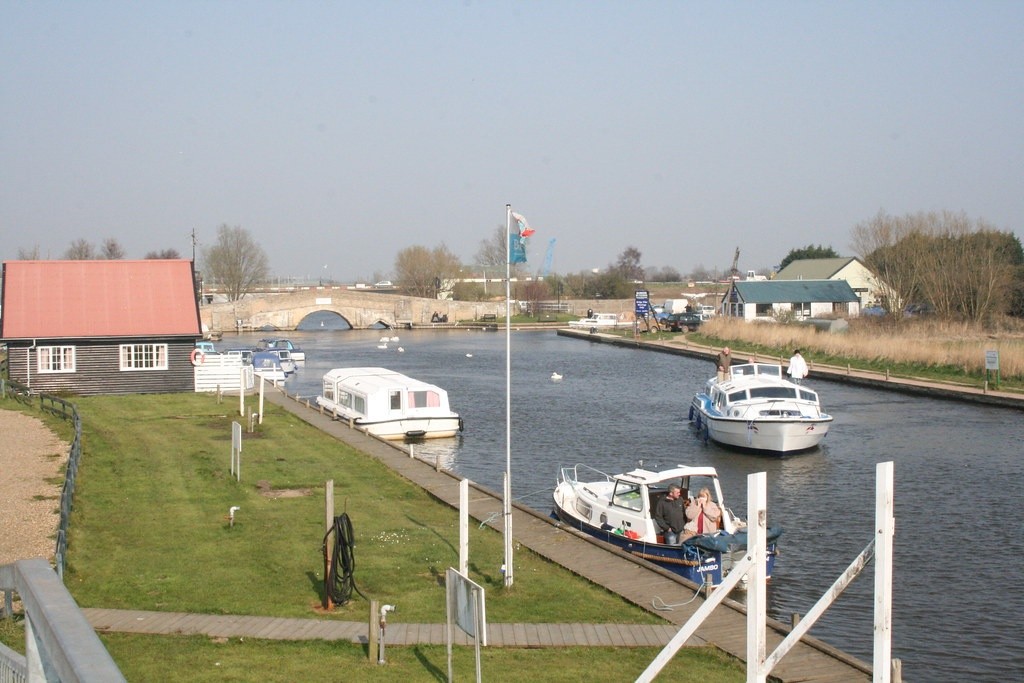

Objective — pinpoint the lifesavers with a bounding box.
[190,347,205,366]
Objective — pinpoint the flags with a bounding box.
[510,234,527,264]
[510,211,536,239]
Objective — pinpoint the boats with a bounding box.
[552,463,779,591]
[568,313,641,329]
[688,362,834,454]
[314,367,464,441]
[196,338,306,381]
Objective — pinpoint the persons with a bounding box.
[787,350,809,385]
[713,347,732,383]
[683,487,720,534]
[654,484,688,545]
[743,358,762,375]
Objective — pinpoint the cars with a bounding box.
[649,299,715,334]
[372,280,393,289]
